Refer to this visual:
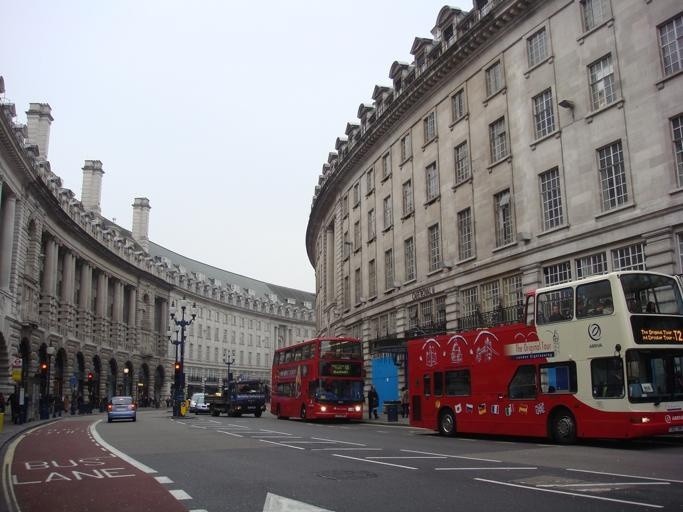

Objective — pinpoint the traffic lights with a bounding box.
[87,372,93,385]
[174,362,180,374]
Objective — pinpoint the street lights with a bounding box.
[169,294,200,419]
[222,349,236,381]
[43,338,55,418]
[122,367,129,395]
[166,324,187,383]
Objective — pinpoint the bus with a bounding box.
[402,267,683,445]
[269,336,365,421]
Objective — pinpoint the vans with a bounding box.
[188,393,209,413]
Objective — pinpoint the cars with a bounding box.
[193,396,211,414]
[105,393,137,423]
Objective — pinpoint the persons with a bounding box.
[550,293,656,321]
[401,387,409,419]
[134,393,175,408]
[0,386,109,426]
[368,385,379,419]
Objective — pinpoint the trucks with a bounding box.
[203,378,267,418]
